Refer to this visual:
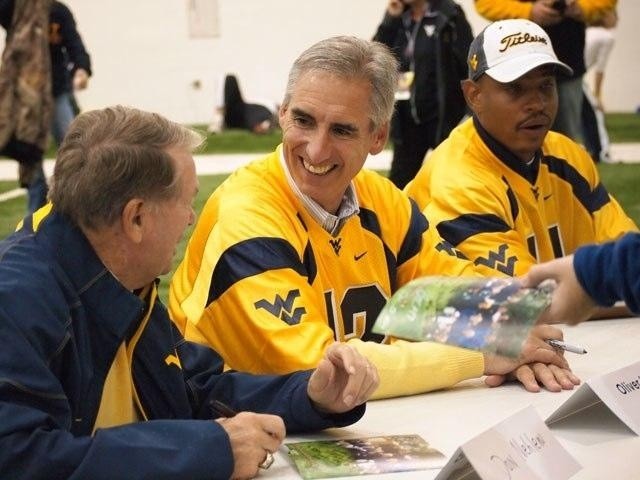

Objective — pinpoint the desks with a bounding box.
[257,316,640,480]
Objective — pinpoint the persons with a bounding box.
[400,17,635,273]
[206,74,279,136]
[582,23,623,164]
[368,0,474,190]
[476,0,620,159]
[1,104,382,479]
[167,31,581,407]
[0,0,93,215]
[520,230,639,327]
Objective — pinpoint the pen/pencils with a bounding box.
[545,339,587,354]
[205,400,291,455]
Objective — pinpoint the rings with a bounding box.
[258,448,274,469]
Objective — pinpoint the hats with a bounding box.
[466,18,573,86]
